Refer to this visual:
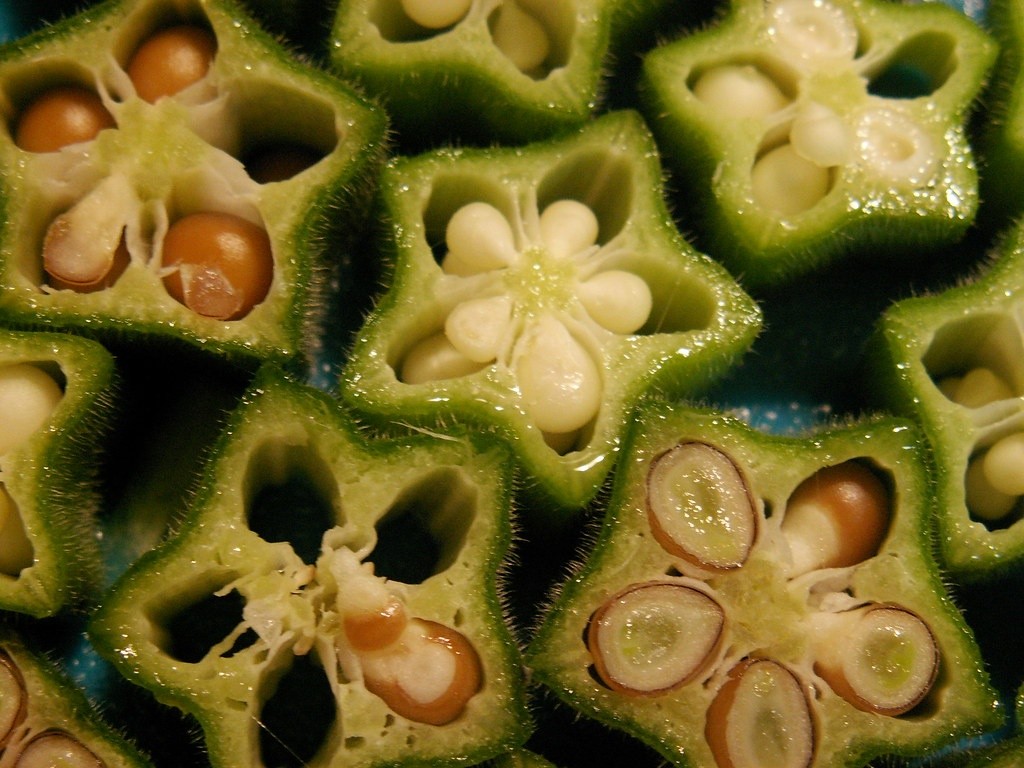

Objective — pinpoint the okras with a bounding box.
[0,0,1024,768]
